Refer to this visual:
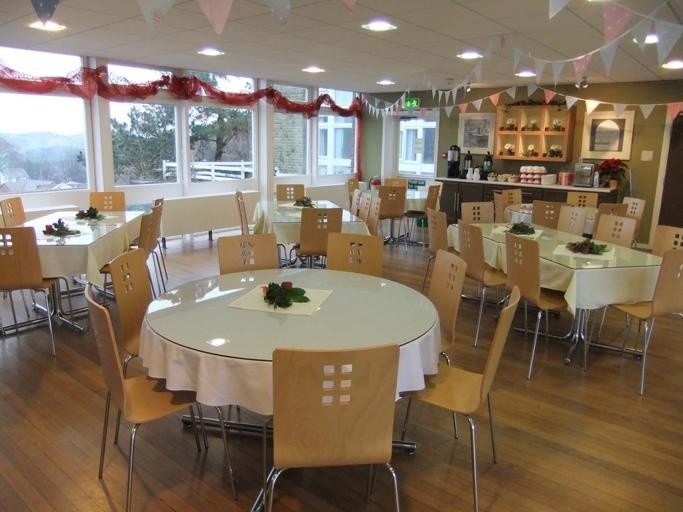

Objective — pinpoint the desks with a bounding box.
[436,176,620,229]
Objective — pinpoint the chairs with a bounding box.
[377,186,404,247]
[0,228,73,356]
[566,191,599,210]
[89,192,126,212]
[404,185,441,246]
[599,251,683,394]
[276,184,305,203]
[399,286,521,512]
[622,197,646,246]
[348,189,360,217]
[420,207,462,294]
[359,192,371,224]
[264,343,401,512]
[366,197,381,236]
[594,214,636,249]
[505,234,567,381]
[84,282,237,512]
[426,249,467,368]
[460,200,493,224]
[151,203,168,291]
[456,219,506,347]
[345,178,359,209]
[493,189,521,226]
[596,203,628,239]
[384,177,407,186]
[325,232,384,278]
[218,234,280,274]
[109,249,208,452]
[0,197,27,227]
[237,193,263,236]
[292,207,343,268]
[140,210,157,300]
[531,200,564,230]
[557,206,586,237]
[651,223,683,262]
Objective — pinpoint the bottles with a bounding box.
[593,171,600,188]
[465,168,473,180]
[483,151,493,171]
[473,168,481,180]
[464,150,473,170]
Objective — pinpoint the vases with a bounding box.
[609,178,621,190]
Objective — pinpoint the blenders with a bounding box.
[447,144,460,179]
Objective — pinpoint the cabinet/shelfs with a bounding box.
[494,106,575,163]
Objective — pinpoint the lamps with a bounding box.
[302,67,327,75]
[376,80,398,87]
[27,20,66,35]
[360,22,396,33]
[457,53,482,62]
[197,48,225,60]
[515,72,536,79]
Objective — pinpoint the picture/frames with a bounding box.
[459,113,495,155]
[581,112,635,161]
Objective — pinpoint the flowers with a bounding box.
[596,158,630,182]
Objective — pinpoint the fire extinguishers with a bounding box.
[368,176,380,190]
